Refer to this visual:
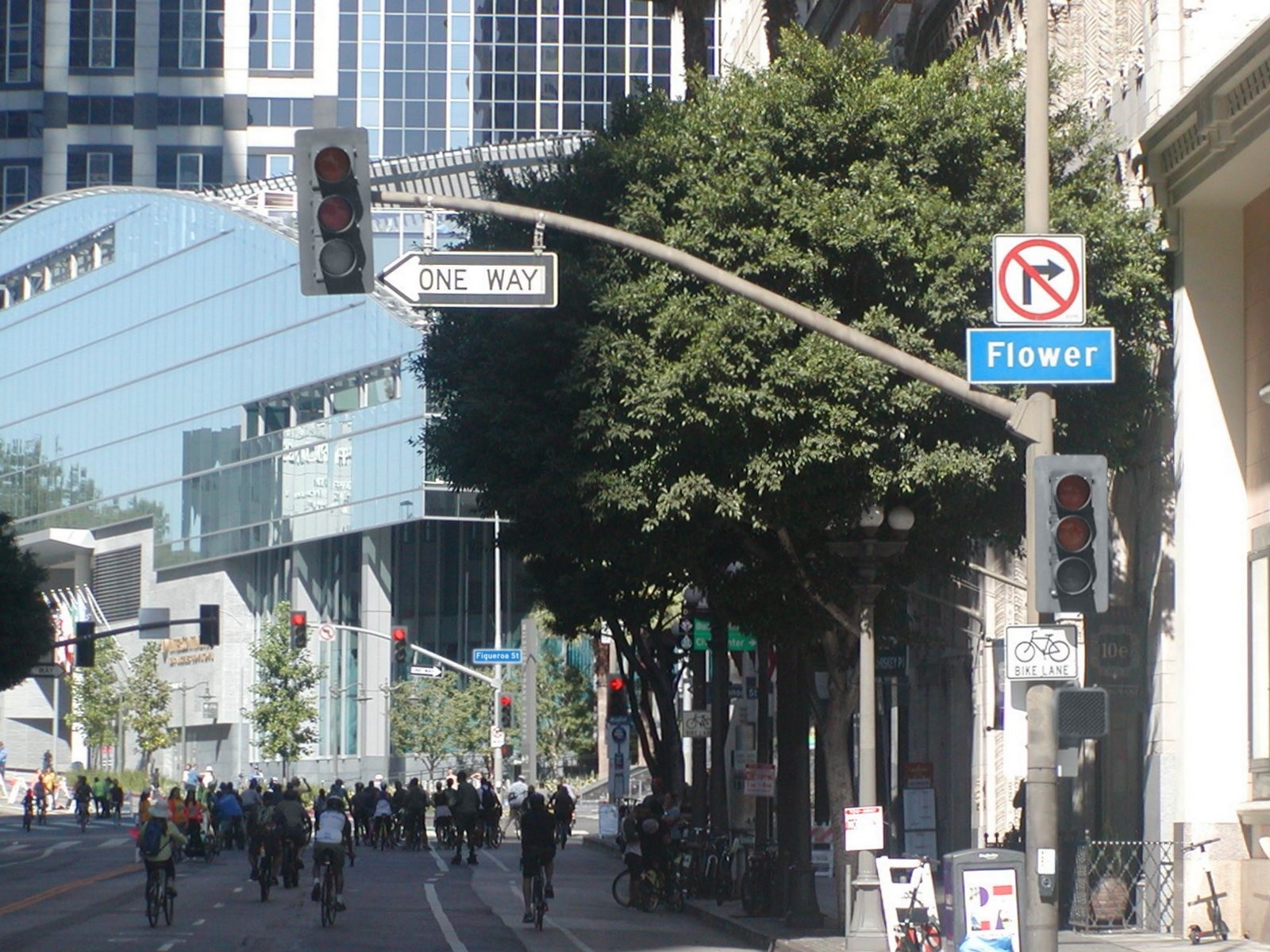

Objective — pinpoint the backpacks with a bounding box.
[140,820,165,857]
[255,804,280,842]
[478,785,495,808]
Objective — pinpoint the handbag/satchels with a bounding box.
[614,836,628,853]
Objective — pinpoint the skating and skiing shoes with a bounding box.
[450,854,463,865]
[468,853,480,865]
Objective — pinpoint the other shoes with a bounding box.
[237,845,245,850]
[222,844,233,851]
[144,906,159,916]
[164,884,178,897]
[336,902,348,911]
[270,875,280,886]
[250,869,260,882]
[522,912,534,923]
[627,901,648,912]
[545,884,554,900]
[312,881,321,902]
[296,858,305,870]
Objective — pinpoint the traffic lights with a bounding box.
[1034,454,1107,614]
[390,625,409,662]
[289,610,307,648]
[294,126,374,296]
[606,673,627,717]
[497,692,513,728]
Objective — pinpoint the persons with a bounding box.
[22,788,34,828]
[72,774,124,821]
[41,750,53,772]
[311,795,354,911]
[618,777,682,910]
[136,799,189,913]
[519,793,556,924]
[0,741,7,779]
[137,766,580,880]
[32,775,52,813]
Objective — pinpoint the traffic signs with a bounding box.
[410,664,444,679]
[374,251,559,308]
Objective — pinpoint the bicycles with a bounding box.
[171,819,222,865]
[252,812,313,903]
[315,848,355,928]
[548,806,576,850]
[357,811,522,852]
[610,824,780,919]
[78,802,87,834]
[24,792,52,833]
[145,843,186,928]
[112,801,120,828]
[520,857,553,932]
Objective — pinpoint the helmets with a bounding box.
[327,795,345,808]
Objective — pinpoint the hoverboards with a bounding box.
[1180,837,1231,944]
[891,855,942,952]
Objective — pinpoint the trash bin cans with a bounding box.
[943,848,1027,952]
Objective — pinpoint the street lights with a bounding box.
[820,499,920,952]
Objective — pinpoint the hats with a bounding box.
[292,774,304,783]
[205,765,214,772]
[147,799,173,818]
